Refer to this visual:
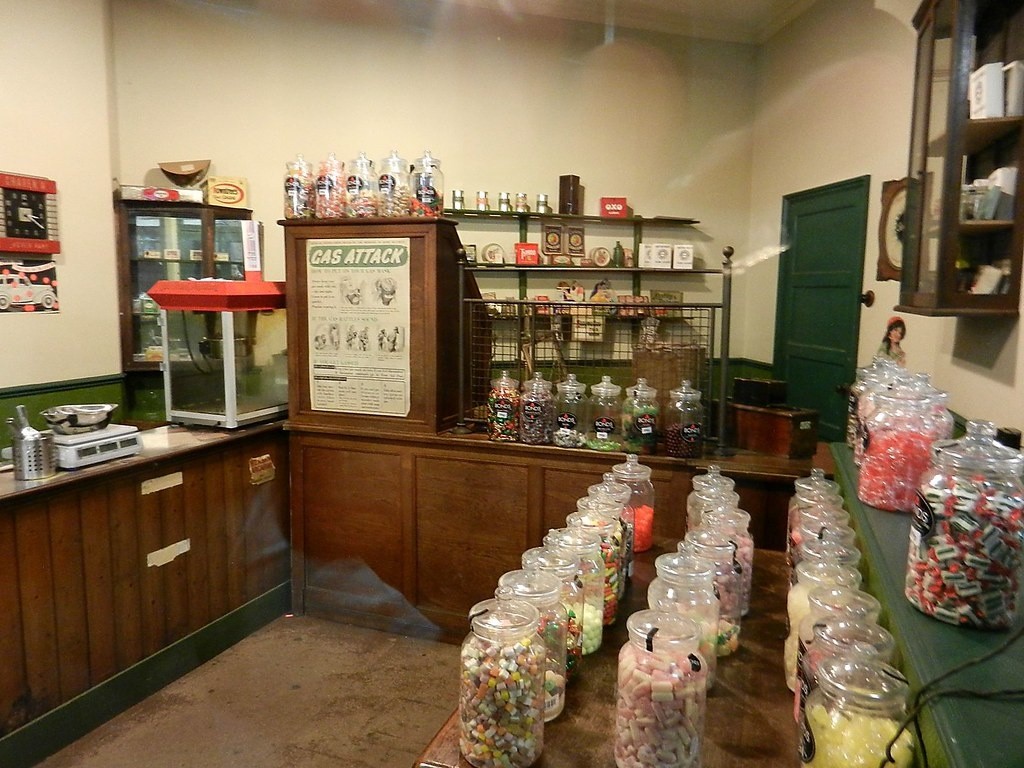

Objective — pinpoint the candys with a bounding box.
[284,166,444,217]
[486,387,703,459]
[905,473,1024,631]
[460,505,914,768]
[853,396,949,512]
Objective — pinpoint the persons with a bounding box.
[878,316,906,365]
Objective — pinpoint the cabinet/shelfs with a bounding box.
[115,197,259,374]
[893,0,1024,321]
[442,207,699,366]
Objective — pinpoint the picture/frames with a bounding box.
[875,172,932,286]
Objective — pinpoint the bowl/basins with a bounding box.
[39,403,119,435]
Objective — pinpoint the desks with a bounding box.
[411,533,800,767]
[824,438,1024,768]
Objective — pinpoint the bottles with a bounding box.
[282,149,546,220]
[458,354,1024,768]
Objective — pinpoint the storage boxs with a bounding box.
[705,399,822,459]
